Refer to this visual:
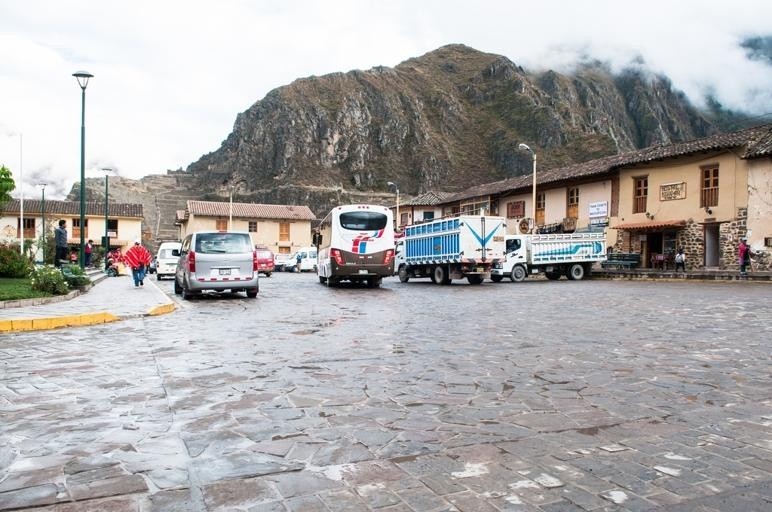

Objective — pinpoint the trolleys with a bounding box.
[749,248,771,272]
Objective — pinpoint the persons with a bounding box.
[85,240,93,266]
[650,249,686,272]
[297,254,302,274]
[739,237,754,271]
[55,220,68,267]
[107,242,150,286]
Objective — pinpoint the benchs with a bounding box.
[58,258,91,291]
[598,251,641,272]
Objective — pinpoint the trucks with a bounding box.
[394,214,505,285]
[490,231,609,282]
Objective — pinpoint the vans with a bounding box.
[255,244,275,279]
[283,247,318,273]
[174,230,261,300]
[156,242,183,280]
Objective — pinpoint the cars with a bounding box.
[150,259,158,274]
[274,252,293,272]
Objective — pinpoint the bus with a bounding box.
[312,204,395,288]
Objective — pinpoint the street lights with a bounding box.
[517,142,537,233]
[6,130,25,256]
[71,70,94,267]
[39,181,52,261]
[100,166,116,269]
[386,181,400,228]
[222,177,233,231]
